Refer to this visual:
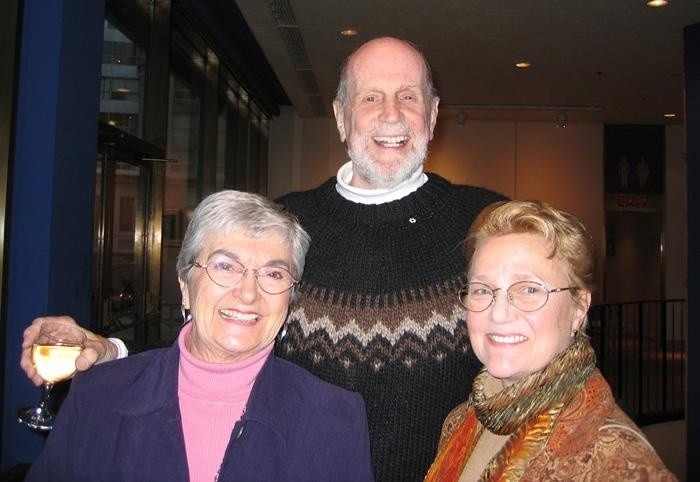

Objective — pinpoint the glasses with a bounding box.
[456,275,579,315]
[187,249,302,297]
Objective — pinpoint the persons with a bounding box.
[23,188,373,481]
[17,34,514,481]
[422,196,681,482]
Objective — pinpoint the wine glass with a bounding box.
[15,320,88,431]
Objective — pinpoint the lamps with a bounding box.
[439,103,603,128]
